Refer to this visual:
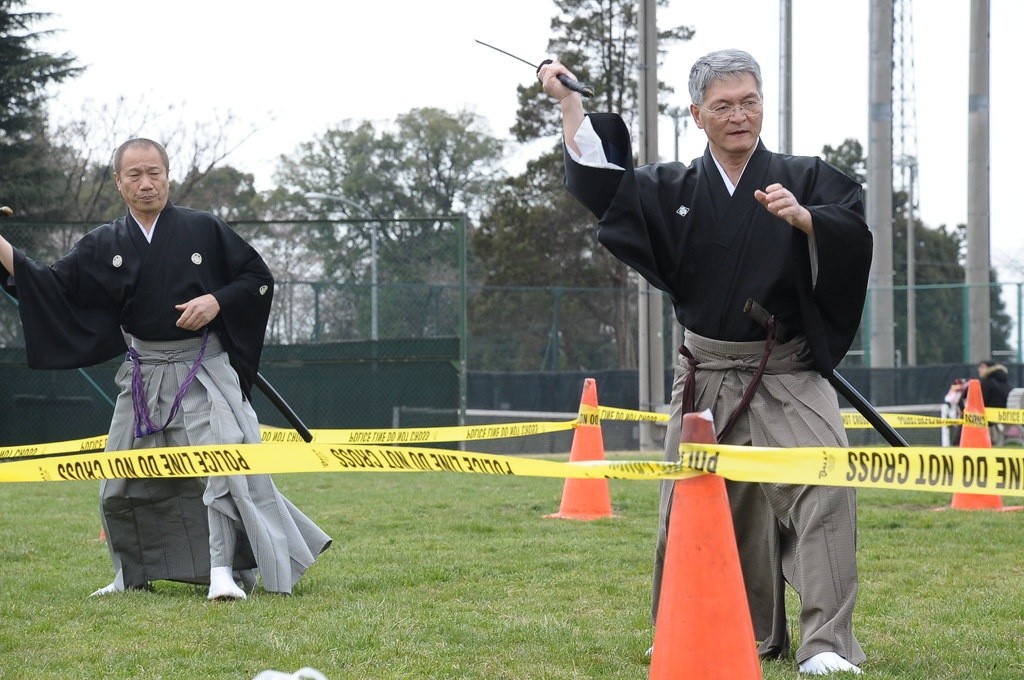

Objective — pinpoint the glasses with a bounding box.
[696,94,764,120]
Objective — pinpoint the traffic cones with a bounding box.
[933,380,1024,512]
[542,378,621,522]
[647,415,762,680]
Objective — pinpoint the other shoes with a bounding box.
[207,566,247,601]
[87,581,117,598]
[800,652,866,676]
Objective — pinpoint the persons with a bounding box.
[538,50,873,675]
[964,360,1007,426]
[0,138,331,599]
